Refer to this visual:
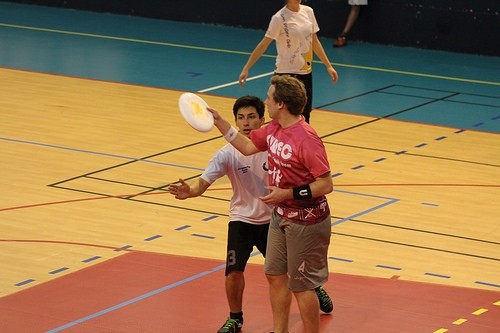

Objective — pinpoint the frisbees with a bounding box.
[178,92,214,132]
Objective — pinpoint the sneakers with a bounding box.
[315,286,333,313]
[333,38,347,47]
[217,317,243,333]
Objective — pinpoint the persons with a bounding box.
[239,0,338,124]
[333,0,368,48]
[168,75,334,333]
